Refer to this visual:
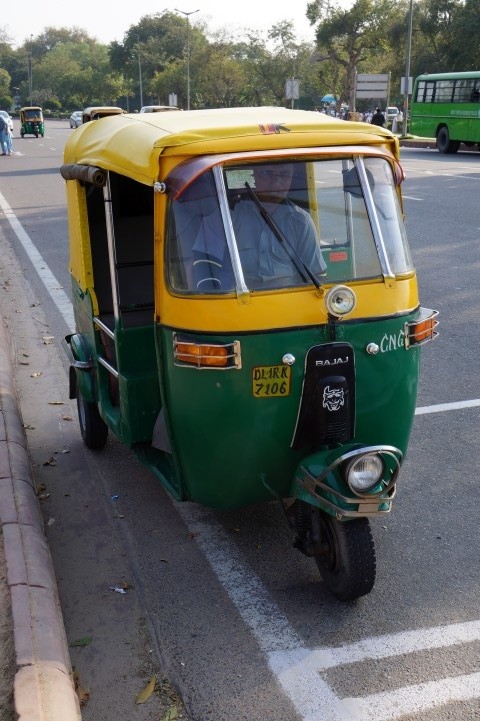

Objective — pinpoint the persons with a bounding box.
[0,115,13,156]
[324,102,404,134]
[152,165,329,450]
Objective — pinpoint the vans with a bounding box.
[386,107,399,120]
[140,105,182,116]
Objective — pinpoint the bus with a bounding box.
[409,71,480,155]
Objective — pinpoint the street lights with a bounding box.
[174,8,201,110]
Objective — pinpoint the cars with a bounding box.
[69,111,83,128]
[0,110,14,131]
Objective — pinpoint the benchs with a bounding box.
[89,216,157,406]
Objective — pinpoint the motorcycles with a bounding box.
[82,106,126,124]
[19,106,44,138]
[63,105,440,599]
